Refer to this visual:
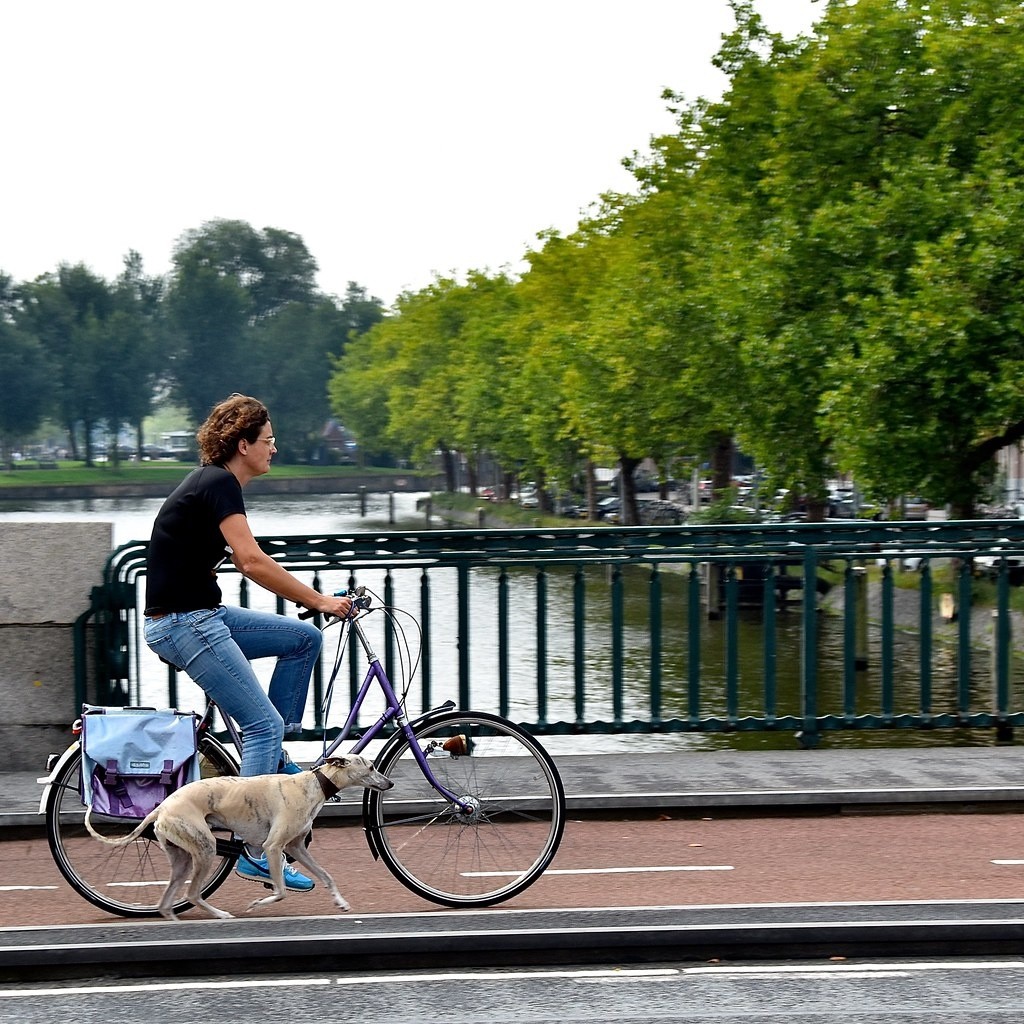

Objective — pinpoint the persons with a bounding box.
[143,393,361,893]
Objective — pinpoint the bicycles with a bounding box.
[37,585,568,909]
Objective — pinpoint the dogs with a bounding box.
[84,754,394,921]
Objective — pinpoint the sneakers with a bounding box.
[234,843,315,893]
[276,747,306,776]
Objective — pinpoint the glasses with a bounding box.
[257,436,276,449]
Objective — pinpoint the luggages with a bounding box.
[79,713,202,825]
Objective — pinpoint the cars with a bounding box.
[78,440,169,461]
[481,465,1024,581]
